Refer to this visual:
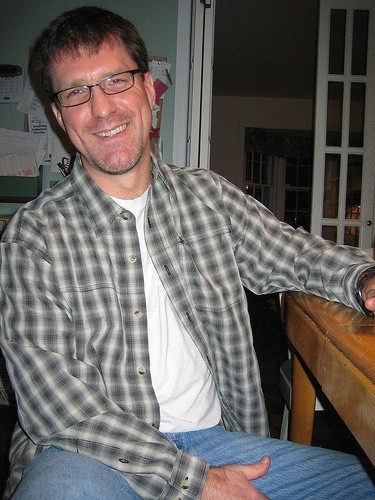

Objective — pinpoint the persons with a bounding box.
[0,6,375,500]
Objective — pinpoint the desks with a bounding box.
[280,288,375,470]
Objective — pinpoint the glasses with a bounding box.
[51,68,143,109]
